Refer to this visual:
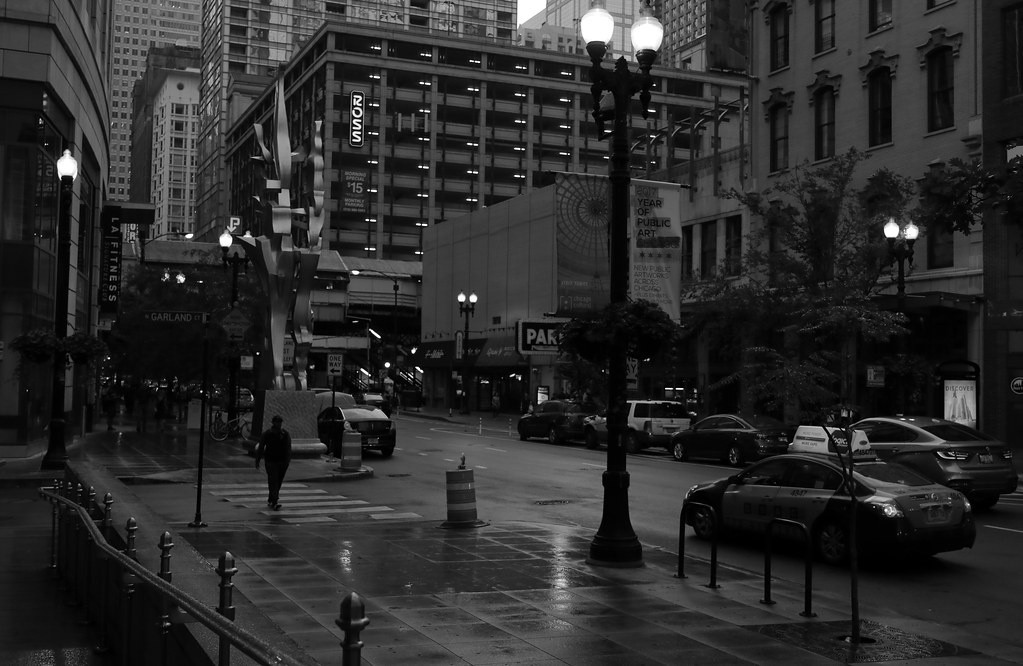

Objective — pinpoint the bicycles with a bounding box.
[210,409,254,442]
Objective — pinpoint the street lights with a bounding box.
[39,150,79,472]
[457,288,479,416]
[352,268,398,409]
[577,0,664,565]
[219,228,252,443]
[139,231,194,290]
[882,216,920,313]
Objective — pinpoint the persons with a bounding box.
[256,416,292,510]
[99,378,192,432]
[492,391,533,417]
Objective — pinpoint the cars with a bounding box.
[517,402,596,445]
[668,414,796,469]
[353,391,392,418]
[846,414,1019,513]
[189,382,255,411]
[684,454,976,574]
[317,405,396,459]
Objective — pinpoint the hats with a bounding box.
[273,416,283,422]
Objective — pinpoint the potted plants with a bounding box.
[61,331,110,371]
[615,300,677,369]
[556,312,610,362]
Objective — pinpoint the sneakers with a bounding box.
[268,502,277,507]
[273,505,282,510]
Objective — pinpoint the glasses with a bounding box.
[273,421,282,423]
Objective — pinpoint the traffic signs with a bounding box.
[327,354,342,376]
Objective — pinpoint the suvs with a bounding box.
[582,399,692,456]
